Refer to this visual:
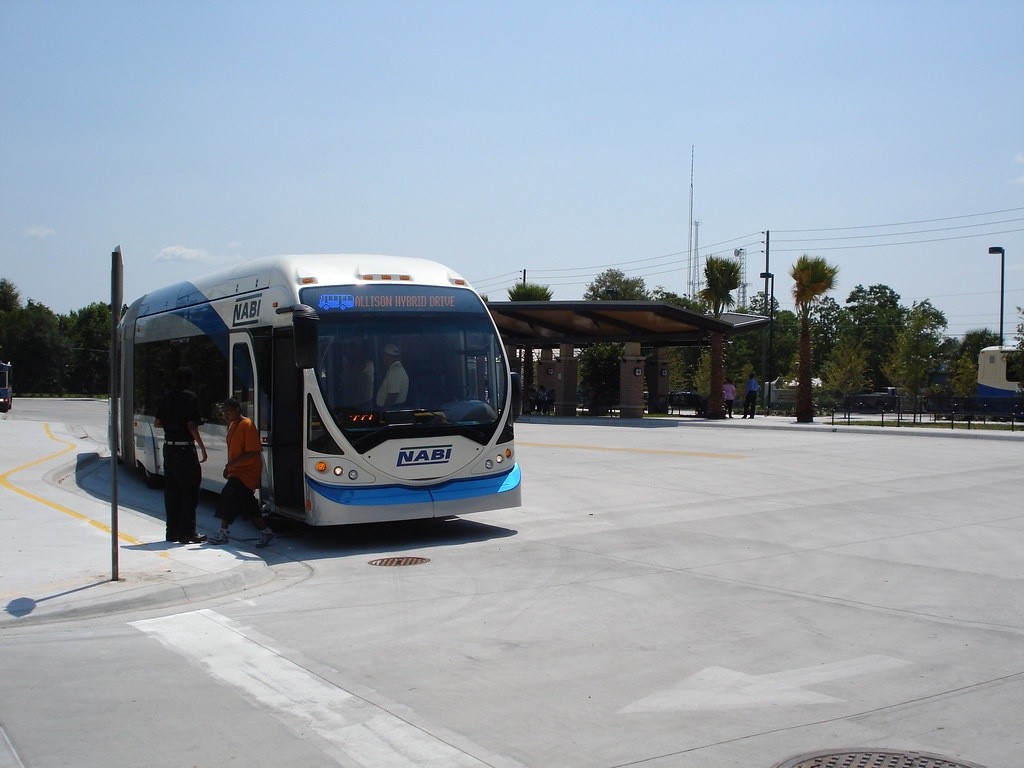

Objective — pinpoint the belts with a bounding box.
[164,440,194,445]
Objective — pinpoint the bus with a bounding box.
[974,347,1024,422]
[0,363,13,412]
[121,252,525,528]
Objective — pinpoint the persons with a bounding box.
[527,383,539,412]
[377,344,409,417]
[208,393,276,548]
[689,384,699,399]
[725,378,736,418]
[535,385,546,415]
[741,373,758,419]
[154,366,208,544]
[543,386,553,414]
[669,386,682,404]
[340,340,372,407]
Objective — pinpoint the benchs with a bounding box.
[669,396,704,416]
[580,399,617,417]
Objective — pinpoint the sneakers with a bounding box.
[208,529,230,545]
[256,528,276,547]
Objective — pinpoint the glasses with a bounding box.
[222,408,237,416]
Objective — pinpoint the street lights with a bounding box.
[988,245,1005,346]
[759,272,774,413]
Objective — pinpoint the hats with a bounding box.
[384,344,402,356]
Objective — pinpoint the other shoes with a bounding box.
[180,532,207,544]
[742,416,746,418]
[166,531,182,542]
[729,416,733,418]
[748,416,754,419]
